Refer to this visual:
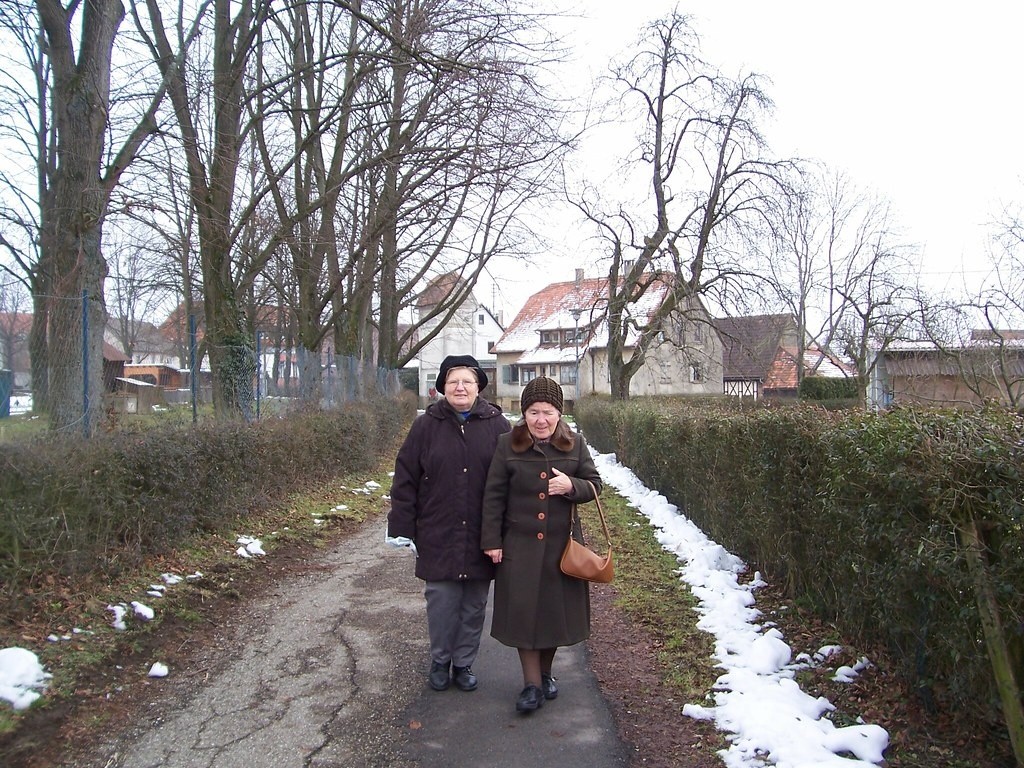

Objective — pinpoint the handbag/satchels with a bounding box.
[560,481,614,583]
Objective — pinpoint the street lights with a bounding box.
[567,309,585,432]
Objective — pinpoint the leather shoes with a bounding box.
[428,658,451,690]
[542,675,560,699]
[452,665,478,690]
[515,685,545,712]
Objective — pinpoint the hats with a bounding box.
[521,376,563,416]
[435,355,488,396]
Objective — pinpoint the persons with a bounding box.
[385,355,512,691]
[479,375,602,711]
